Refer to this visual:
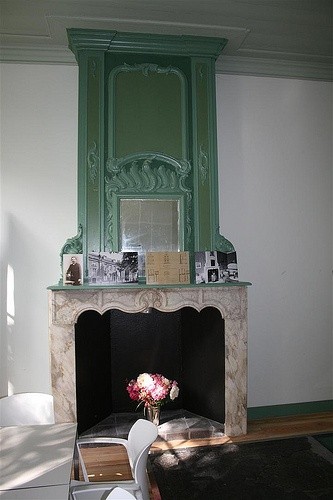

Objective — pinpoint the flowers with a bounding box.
[127,371,179,405]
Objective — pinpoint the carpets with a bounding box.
[148,431,333,500]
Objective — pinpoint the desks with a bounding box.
[0,421,79,491]
[1,486,68,499]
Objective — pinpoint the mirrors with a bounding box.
[106,151,193,251]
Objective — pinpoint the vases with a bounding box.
[147,403,159,424]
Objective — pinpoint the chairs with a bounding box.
[0,392,56,424]
[71,419,157,499]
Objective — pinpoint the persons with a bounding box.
[66,256,81,286]
